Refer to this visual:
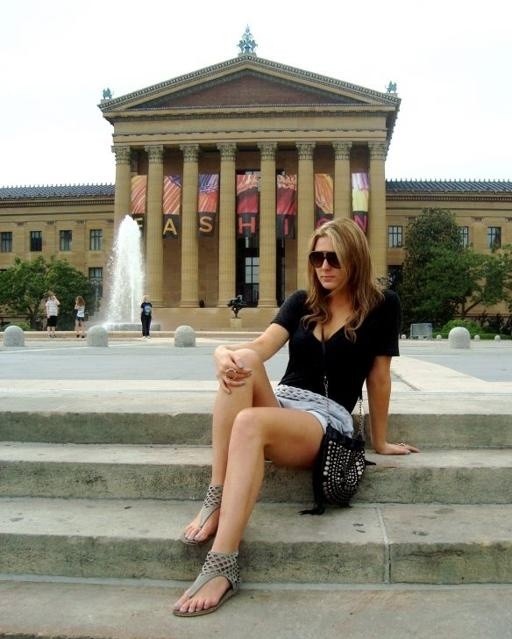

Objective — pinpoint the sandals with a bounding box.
[171,552,241,617]
[180,484,224,546]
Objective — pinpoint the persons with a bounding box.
[74,296,86,338]
[140,295,153,339]
[45,295,61,338]
[169,217,419,618]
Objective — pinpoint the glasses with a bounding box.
[309,251,341,269]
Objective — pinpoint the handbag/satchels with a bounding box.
[311,422,367,509]
